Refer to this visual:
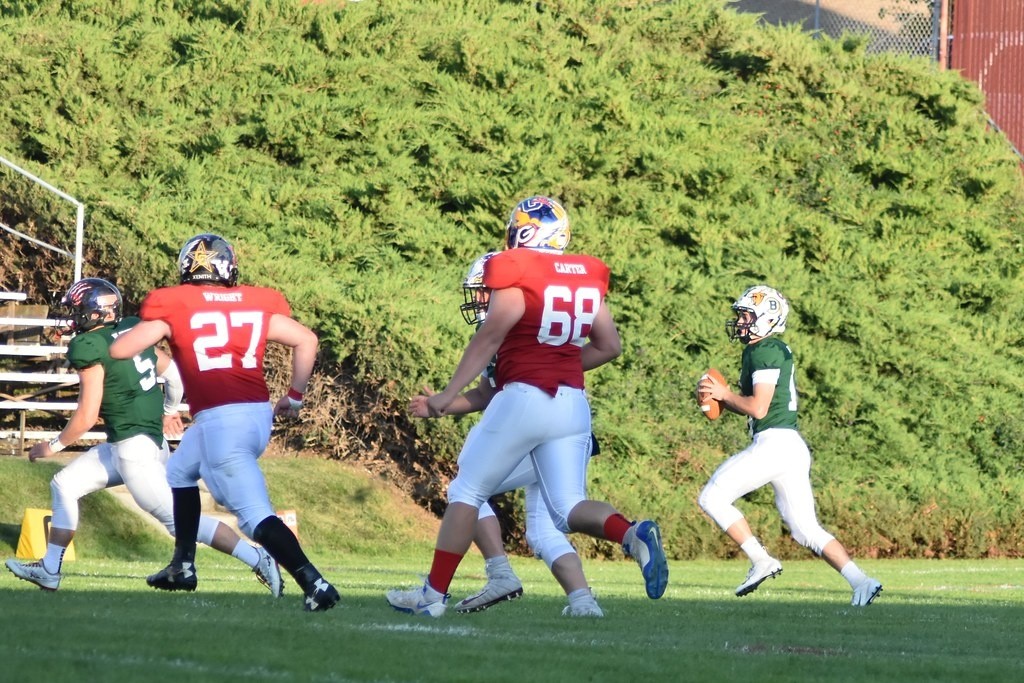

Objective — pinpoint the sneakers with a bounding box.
[561,594,606,618]
[251,546,285,598]
[454,569,523,614]
[5,559,62,592]
[735,557,782,597]
[849,577,882,606]
[146,543,196,592]
[293,563,340,611]
[386,574,452,618]
[622,520,669,599]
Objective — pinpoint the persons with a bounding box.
[697,286,882,607]
[109,235,341,613]
[5,278,285,599]
[385,196,669,618]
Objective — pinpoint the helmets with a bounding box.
[460,250,503,325]
[725,286,789,344]
[61,278,123,335]
[505,196,570,255]
[175,233,238,287]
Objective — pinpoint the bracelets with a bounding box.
[288,388,302,401]
[49,438,65,452]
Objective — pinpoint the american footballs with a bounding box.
[698,367,727,421]
